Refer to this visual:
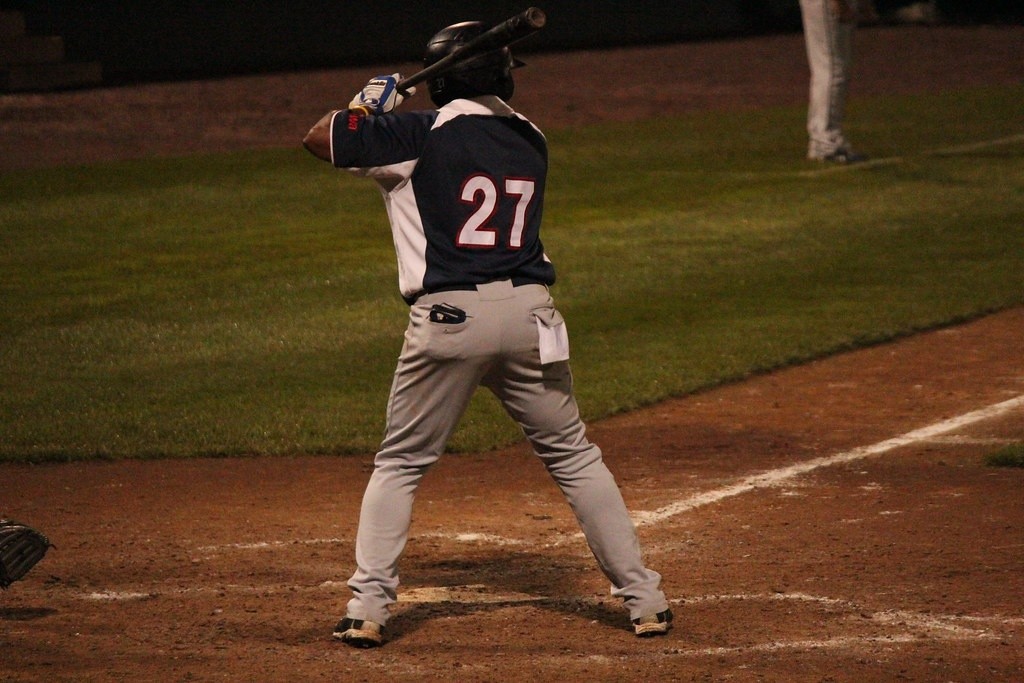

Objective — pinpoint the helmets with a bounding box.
[423,19,530,108]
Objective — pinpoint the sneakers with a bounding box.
[330,615,388,651]
[631,600,676,638]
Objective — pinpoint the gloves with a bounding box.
[355,71,417,115]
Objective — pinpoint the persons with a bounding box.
[304,23,674,644]
[800,0,860,164]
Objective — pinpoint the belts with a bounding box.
[425,275,551,294]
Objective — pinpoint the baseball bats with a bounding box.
[395,5,547,93]
